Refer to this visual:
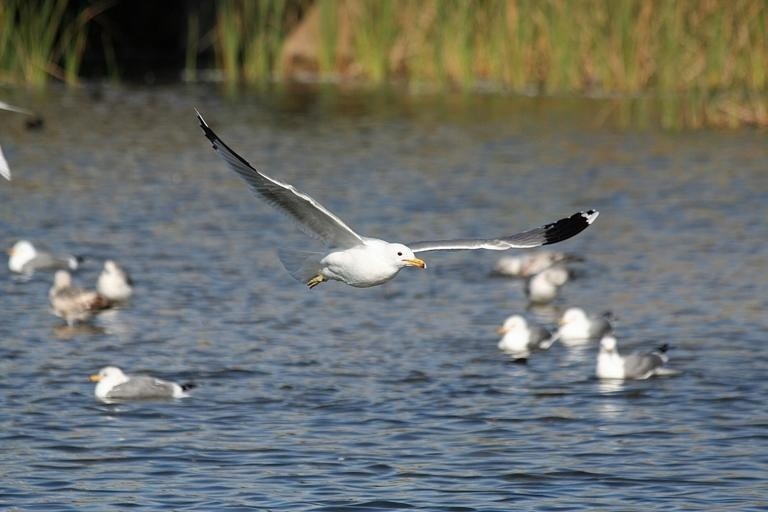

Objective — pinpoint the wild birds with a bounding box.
[3,240,90,276]
[557,307,620,340]
[525,268,575,306]
[47,267,124,329]
[495,313,561,358]
[96,259,135,299]
[493,249,586,278]
[90,366,195,407]
[594,334,686,382]
[191,104,600,290]
